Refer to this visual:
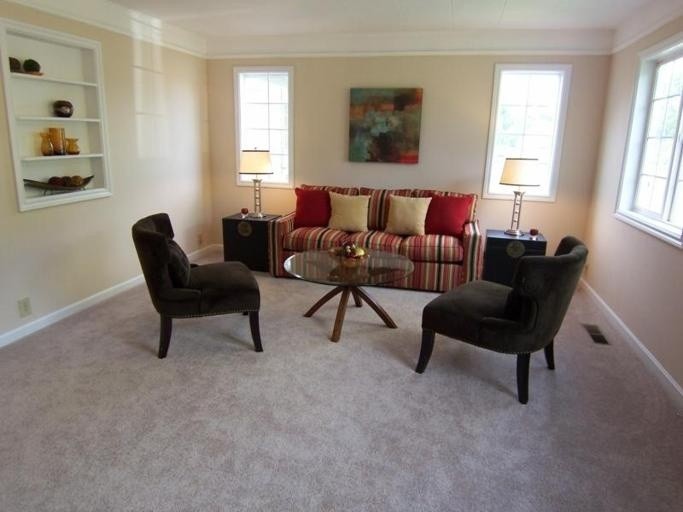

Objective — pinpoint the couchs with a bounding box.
[267,184,483,293]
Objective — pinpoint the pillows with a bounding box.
[293,187,473,237]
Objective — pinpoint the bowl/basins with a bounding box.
[327,246,370,268]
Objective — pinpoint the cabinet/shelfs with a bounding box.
[483,230,547,286]
[222,213,282,272]
[0,16,112,212]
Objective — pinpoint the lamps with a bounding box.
[499,158,541,236]
[239,150,274,218]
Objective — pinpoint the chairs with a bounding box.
[415,235,589,404]
[132,212,264,358]
